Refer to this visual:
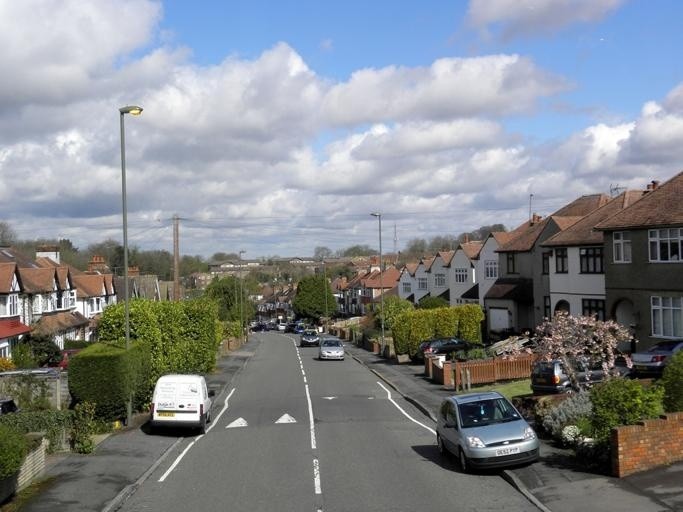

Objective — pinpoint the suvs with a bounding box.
[530,353,634,395]
[420,338,484,362]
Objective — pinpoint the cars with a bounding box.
[46,349,86,373]
[435,391,541,470]
[298,329,322,346]
[244,315,314,335]
[0,400,19,415]
[630,340,683,377]
[317,336,347,361]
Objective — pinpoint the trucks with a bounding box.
[150,375,217,434]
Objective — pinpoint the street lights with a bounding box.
[370,212,387,357]
[119,102,147,425]
[239,249,249,344]
[318,255,329,335]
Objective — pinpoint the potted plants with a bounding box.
[0,423,48,507]
